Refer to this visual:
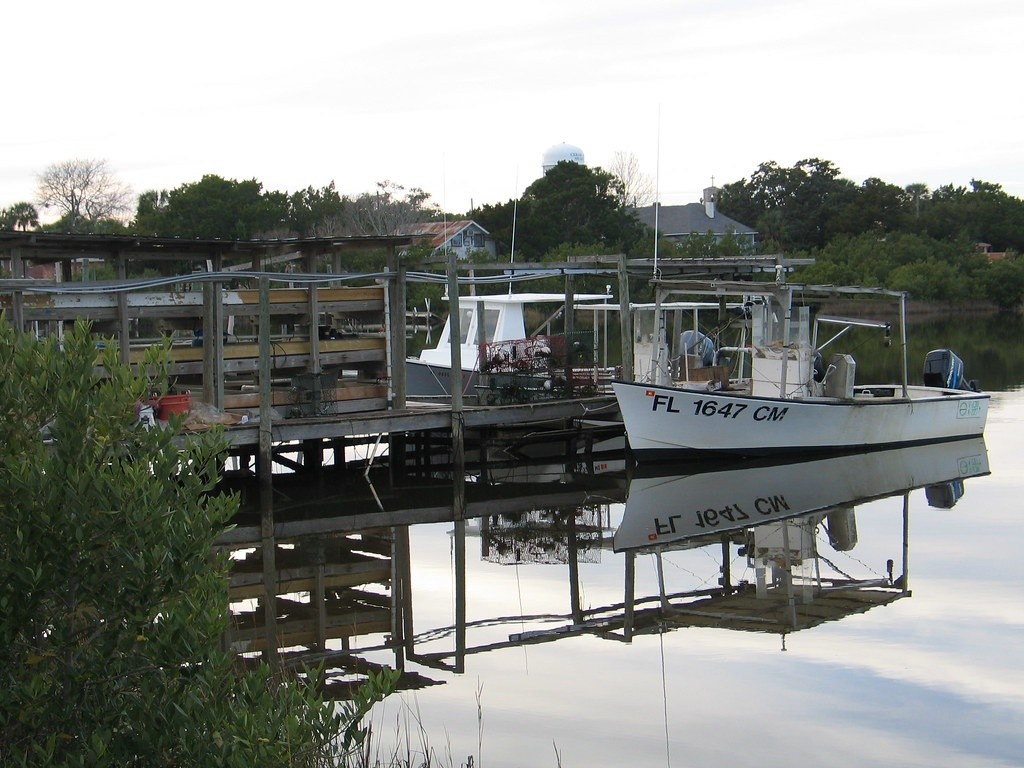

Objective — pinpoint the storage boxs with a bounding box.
[158,402,190,421]
[160,394,191,401]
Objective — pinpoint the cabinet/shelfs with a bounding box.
[1,225,393,417]
[204,515,444,697]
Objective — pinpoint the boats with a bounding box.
[616,304,990,453]
[406,292,622,397]
